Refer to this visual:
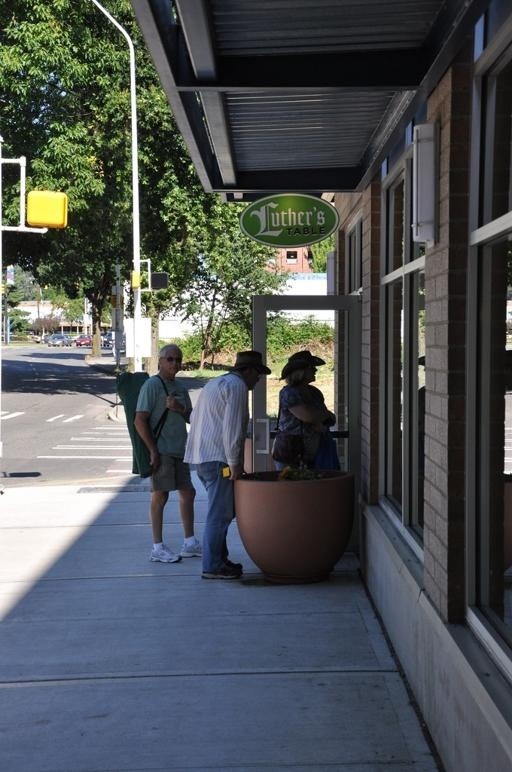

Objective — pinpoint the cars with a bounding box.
[32,332,112,347]
[1,331,18,341]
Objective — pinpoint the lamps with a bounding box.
[409,120,442,249]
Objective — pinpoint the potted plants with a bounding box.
[234,454,354,587]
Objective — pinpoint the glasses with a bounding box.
[167,356,182,362]
[255,367,264,378]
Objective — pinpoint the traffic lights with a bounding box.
[151,272,169,290]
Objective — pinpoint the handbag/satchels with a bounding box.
[117,371,169,478]
[272,385,303,469]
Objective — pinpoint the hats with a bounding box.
[280,351,326,379]
[232,352,270,375]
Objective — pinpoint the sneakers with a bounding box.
[151,545,180,563]
[180,543,203,557]
[201,560,242,578]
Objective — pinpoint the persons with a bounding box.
[133,343,204,563]
[181,351,270,580]
[270,350,340,472]
